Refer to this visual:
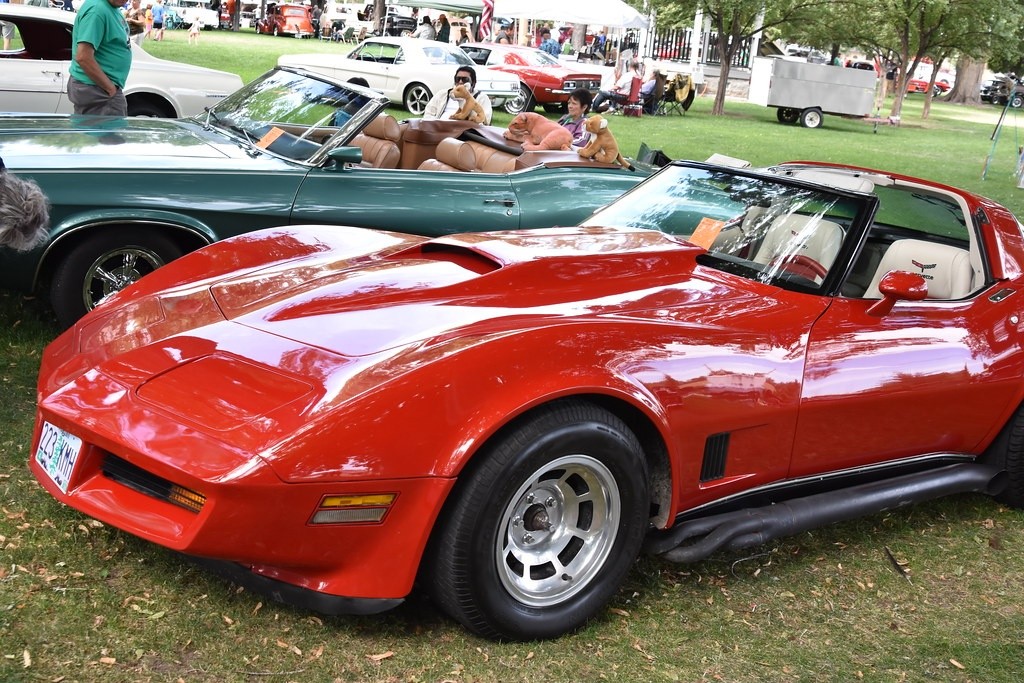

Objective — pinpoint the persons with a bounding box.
[1016,145,1024,188]
[456,28,469,46]
[253,5,262,30]
[592,43,660,113]
[592,30,606,52]
[424,66,492,126]
[70,113,128,145]
[536,23,562,59]
[329,78,370,127]
[411,14,450,44]
[0,0,82,50]
[834,52,852,67]
[0,157,51,252]
[557,88,592,147]
[481,17,514,54]
[312,5,351,42]
[120,0,236,44]
[67,0,132,119]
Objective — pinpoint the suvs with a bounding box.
[0,1,245,120]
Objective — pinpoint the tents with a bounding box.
[380,0,494,57]
[492,0,650,70]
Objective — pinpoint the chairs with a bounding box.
[610,77,643,117]
[294,24,313,39]
[168,9,184,31]
[344,113,401,171]
[637,73,668,116]
[319,29,332,42]
[351,27,368,44]
[662,74,692,117]
[860,237,973,301]
[415,135,477,173]
[336,27,355,44]
[753,213,847,285]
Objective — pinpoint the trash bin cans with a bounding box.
[311,18,320,39]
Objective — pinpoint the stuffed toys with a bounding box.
[577,115,636,172]
[503,112,573,153]
[449,82,485,123]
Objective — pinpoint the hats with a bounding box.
[438,14,445,19]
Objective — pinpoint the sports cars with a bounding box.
[15,155,1024,642]
[0,62,777,338]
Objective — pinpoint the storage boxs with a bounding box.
[624,105,643,117]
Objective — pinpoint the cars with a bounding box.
[558,26,595,46]
[216,0,417,39]
[431,18,473,45]
[786,43,1024,108]
[457,42,603,116]
[162,1,220,32]
[491,17,514,44]
[276,34,523,115]
[655,39,691,59]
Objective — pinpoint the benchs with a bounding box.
[466,139,518,174]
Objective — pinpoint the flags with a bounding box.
[480,0,495,38]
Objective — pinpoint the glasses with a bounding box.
[455,77,469,84]
